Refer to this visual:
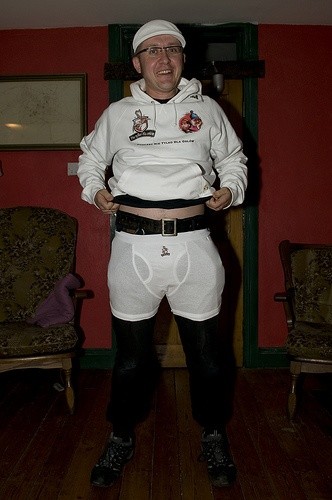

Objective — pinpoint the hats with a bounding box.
[132,19,188,52]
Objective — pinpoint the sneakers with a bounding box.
[196,429,237,487]
[88,428,133,486]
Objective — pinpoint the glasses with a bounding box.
[135,45,182,55]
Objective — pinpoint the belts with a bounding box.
[115,209,211,237]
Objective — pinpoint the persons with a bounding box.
[77,20,248,487]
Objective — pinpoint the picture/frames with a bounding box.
[0,73,88,150]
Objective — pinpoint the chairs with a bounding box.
[0,206,88,415]
[273,239,332,420]
